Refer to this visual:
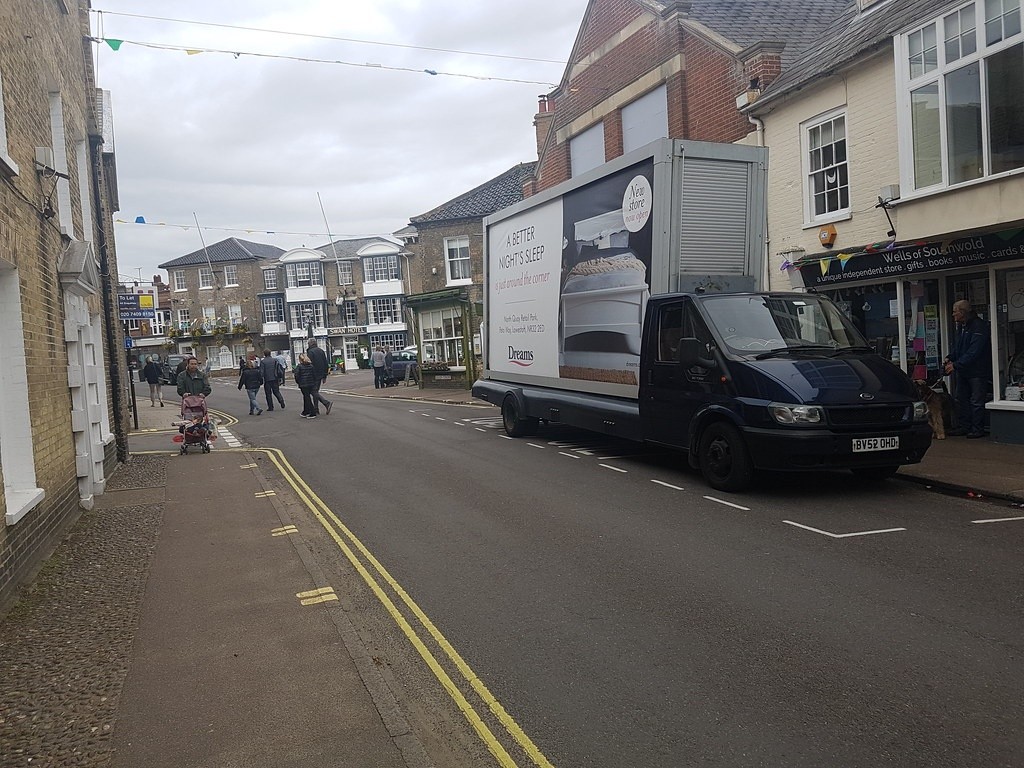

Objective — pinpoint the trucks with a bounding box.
[470,136,937,494]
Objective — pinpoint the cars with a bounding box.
[403,344,463,361]
[158,354,190,386]
[384,351,417,382]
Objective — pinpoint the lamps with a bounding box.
[846,284,885,296]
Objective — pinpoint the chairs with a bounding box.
[661,309,692,360]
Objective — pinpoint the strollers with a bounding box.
[179,394,212,455]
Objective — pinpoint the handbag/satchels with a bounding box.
[275,358,285,378]
[158,377,164,385]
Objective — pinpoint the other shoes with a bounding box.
[151,405,155,407]
[326,401,333,414]
[967,431,986,438]
[256,409,264,415]
[267,408,274,412]
[161,401,164,407]
[281,400,286,409]
[249,412,254,416]
[947,428,970,437]
[300,413,317,418]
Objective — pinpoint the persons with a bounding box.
[260,349,286,411]
[362,348,369,369]
[238,360,264,416]
[175,354,212,414]
[371,344,393,389]
[171,413,212,437]
[238,349,288,386]
[942,299,991,440]
[143,356,164,408]
[294,353,318,419]
[307,338,333,416]
